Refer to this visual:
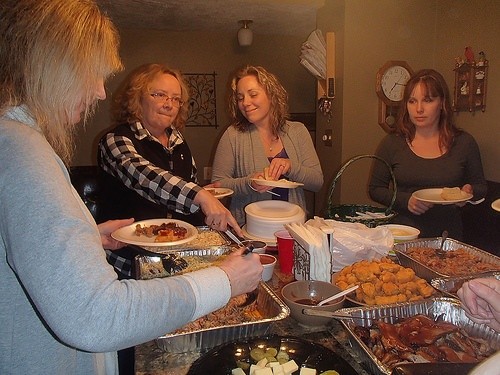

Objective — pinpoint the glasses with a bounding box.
[150,93,184,108]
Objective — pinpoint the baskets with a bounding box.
[320,155,399,228]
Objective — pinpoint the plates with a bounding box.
[111,218,199,247]
[491,198,500,212]
[204,187,234,200]
[411,188,473,204]
[245,200,305,238]
[345,295,369,307]
[184,333,359,375]
[253,178,304,188]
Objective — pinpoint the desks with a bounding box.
[133,252,376,375]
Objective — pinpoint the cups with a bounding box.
[257,253,277,283]
[238,239,267,255]
[272,230,295,274]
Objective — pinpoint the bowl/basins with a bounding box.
[376,223,419,256]
[279,280,346,329]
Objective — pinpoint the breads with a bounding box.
[265,167,278,181]
[439,187,467,200]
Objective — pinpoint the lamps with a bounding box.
[236,19,254,46]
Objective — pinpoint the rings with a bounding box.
[212,222,220,225]
[280,164,285,168]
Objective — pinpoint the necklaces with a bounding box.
[263,137,272,150]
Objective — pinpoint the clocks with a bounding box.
[376,60,416,135]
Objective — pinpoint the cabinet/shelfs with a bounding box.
[285,216,335,284]
[452,61,488,113]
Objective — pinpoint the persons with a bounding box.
[0,0,264,375]
[368,68,488,241]
[210,65,324,229]
[97,63,243,374]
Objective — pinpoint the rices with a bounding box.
[142,254,213,278]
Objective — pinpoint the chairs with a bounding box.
[70,166,129,223]
